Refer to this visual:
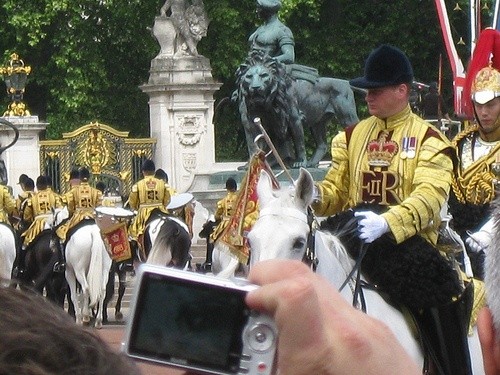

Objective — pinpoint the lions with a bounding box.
[234,49,368,168]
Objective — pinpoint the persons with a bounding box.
[450,66,500,279]
[478,208,500,375]
[161,0,200,57]
[201,177,240,272]
[0,170,63,272]
[309,42,472,375]
[55,168,103,240]
[124,158,170,272]
[238,0,294,170]
[0,258,420,375]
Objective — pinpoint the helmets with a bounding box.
[45,177,52,185]
[37,176,46,187]
[96,182,104,189]
[142,160,154,170]
[349,44,414,87]
[24,178,34,186]
[460,27,500,121]
[19,174,28,183]
[80,169,88,178]
[155,169,168,182]
[225,177,237,189]
[70,171,79,179]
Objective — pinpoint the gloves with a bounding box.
[353,211,390,242]
[466,231,493,253]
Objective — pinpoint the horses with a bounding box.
[245,165,485,375]
[0,205,249,330]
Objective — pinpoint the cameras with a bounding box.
[121,264,278,375]
